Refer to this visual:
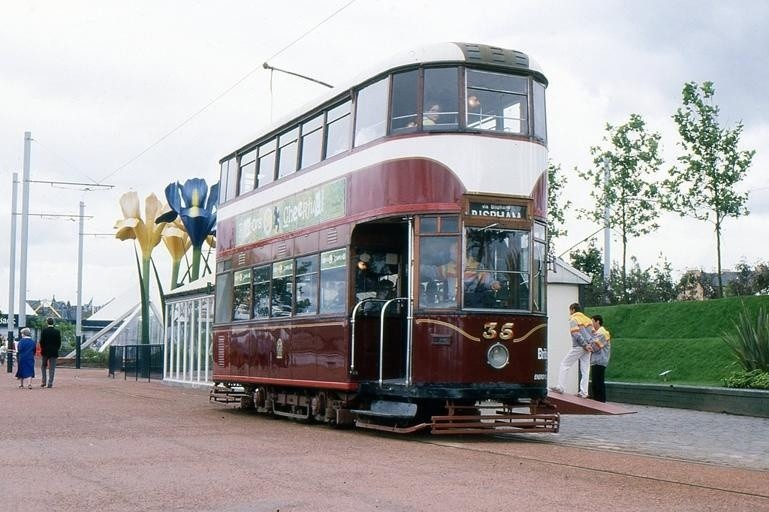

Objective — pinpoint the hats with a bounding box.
[20,327,31,336]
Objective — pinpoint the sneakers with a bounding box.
[549,385,589,398]
[18,383,53,390]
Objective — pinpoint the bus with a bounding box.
[207,43,561,436]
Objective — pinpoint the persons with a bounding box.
[14,326,36,389]
[406,101,442,129]
[0,334,50,368]
[233,240,501,308]
[40,317,61,388]
[547,301,594,398]
[590,315,612,404]
